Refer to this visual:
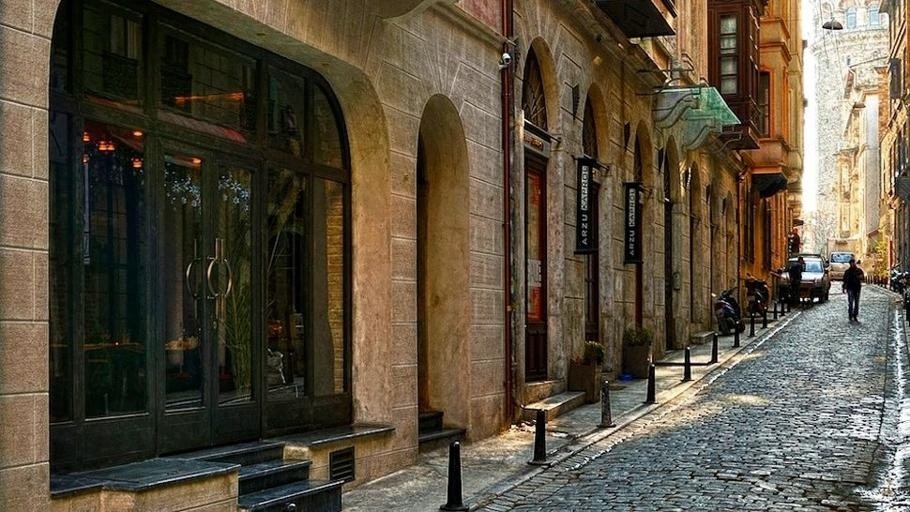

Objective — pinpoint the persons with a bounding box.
[842,259,864,321]
[789,257,804,308]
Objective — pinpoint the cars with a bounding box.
[270,312,304,339]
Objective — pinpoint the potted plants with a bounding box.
[568,340,607,404]
[623,323,654,379]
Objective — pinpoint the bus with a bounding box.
[828,251,862,282]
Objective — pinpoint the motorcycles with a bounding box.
[711,286,748,335]
[741,272,773,319]
[889,267,910,293]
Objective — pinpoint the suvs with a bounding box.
[776,252,832,304]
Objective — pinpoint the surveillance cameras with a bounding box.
[499,53,512,71]
